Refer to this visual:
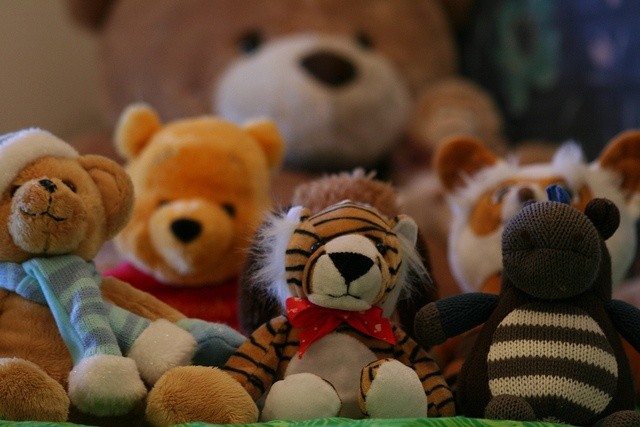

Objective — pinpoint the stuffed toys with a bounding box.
[222,169,455,421]
[0,127,259,427]
[433,130,638,427]
[62,2,503,297]
[90,102,288,339]
[416,198,639,427]
